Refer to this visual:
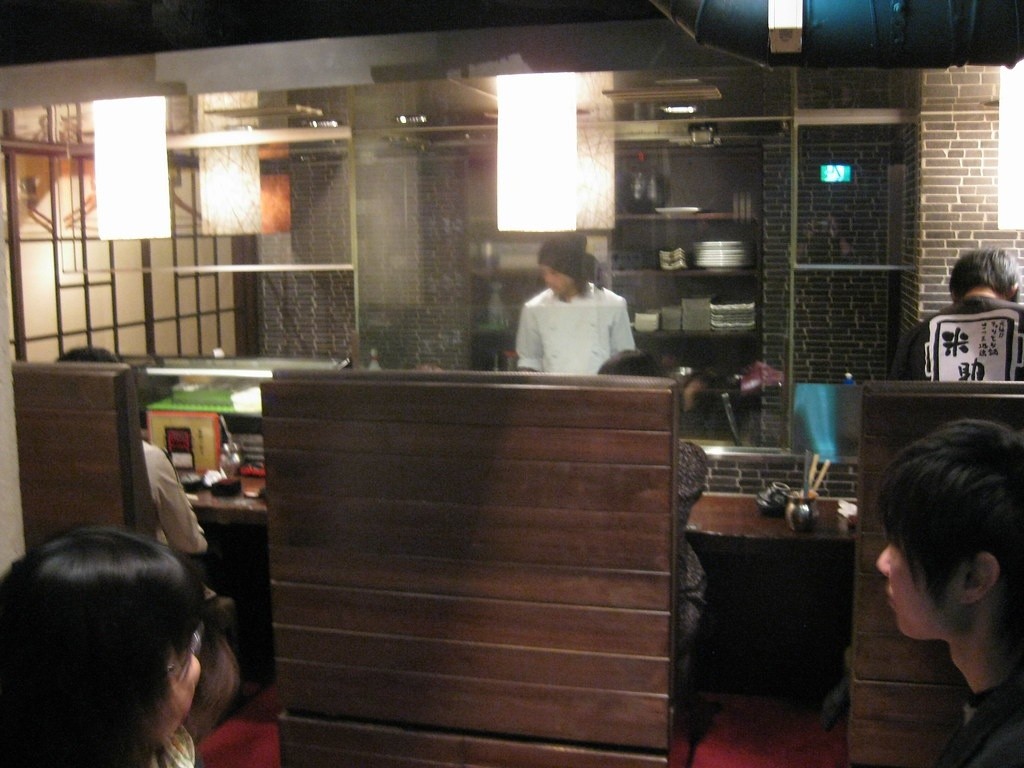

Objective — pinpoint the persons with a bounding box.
[516,232,636,376]
[0,522,288,768]
[873,418,1024,768]
[888,250,1024,383]
[598,350,709,728]
[55,347,224,596]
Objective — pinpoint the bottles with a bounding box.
[648,173,659,203]
[635,172,644,199]
[369,349,378,370]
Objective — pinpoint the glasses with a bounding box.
[168,631,200,687]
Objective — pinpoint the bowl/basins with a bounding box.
[635,312,660,332]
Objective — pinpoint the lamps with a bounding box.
[767,0,803,53]
[688,124,715,144]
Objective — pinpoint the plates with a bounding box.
[658,241,756,271]
[663,298,757,330]
[655,206,699,213]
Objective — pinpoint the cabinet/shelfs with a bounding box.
[454,115,761,447]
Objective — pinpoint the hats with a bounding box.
[539,231,587,279]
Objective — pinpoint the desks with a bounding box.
[685,496,855,697]
[176,468,270,654]
